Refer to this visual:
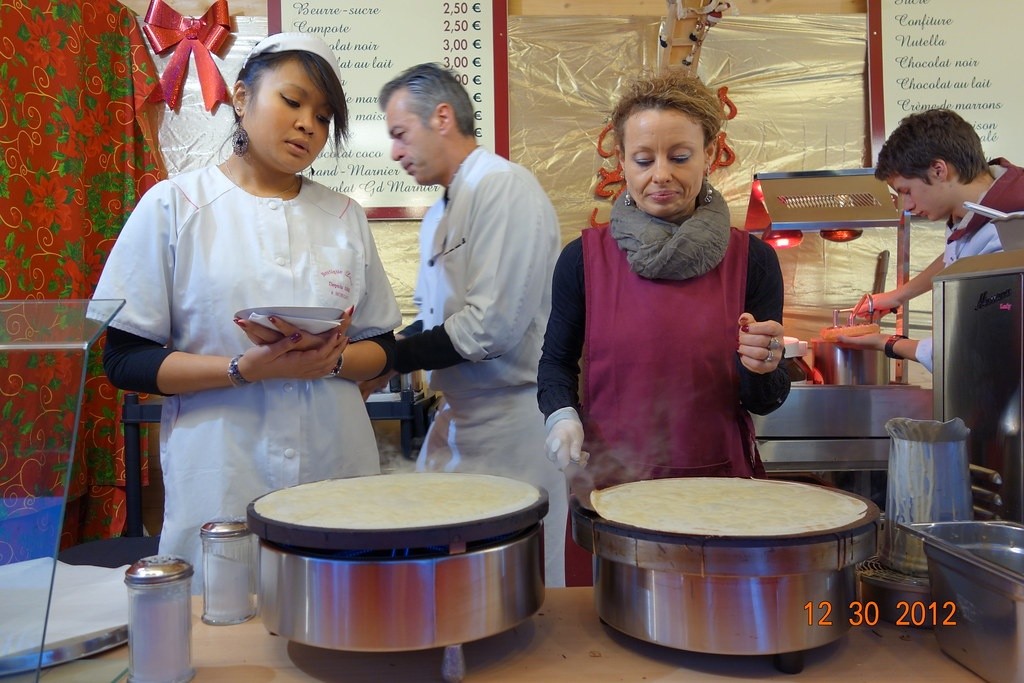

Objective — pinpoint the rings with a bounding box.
[765,349,774,362]
[768,335,780,349]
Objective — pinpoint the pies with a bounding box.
[254,472,540,530]
[588,476,868,536]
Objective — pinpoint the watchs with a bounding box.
[884,333,909,359]
[322,352,343,379]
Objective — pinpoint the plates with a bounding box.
[234,305,344,322]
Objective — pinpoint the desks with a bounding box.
[0,573,990,683]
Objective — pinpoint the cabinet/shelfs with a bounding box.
[120,392,438,538]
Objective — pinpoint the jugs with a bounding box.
[879,417,977,577]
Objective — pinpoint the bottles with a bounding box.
[124,556,195,683]
[200,518,260,625]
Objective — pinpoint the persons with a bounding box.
[535,73,791,503]
[87,30,403,593]
[840,108,1024,374]
[353,62,571,587]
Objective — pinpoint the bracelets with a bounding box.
[228,353,254,385]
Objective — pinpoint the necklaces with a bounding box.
[222,157,299,199]
